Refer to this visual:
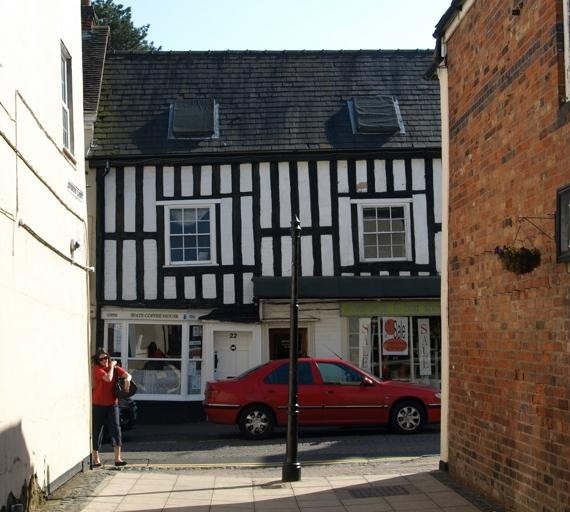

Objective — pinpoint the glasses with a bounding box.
[99,356,108,361]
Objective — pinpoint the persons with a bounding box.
[148,341,165,362]
[92,347,132,466]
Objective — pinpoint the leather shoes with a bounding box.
[115,461,127,466]
[93,460,101,466]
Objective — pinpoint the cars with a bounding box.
[201,343,445,435]
[103,396,143,438]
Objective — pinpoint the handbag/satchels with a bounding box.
[114,378,137,399]
